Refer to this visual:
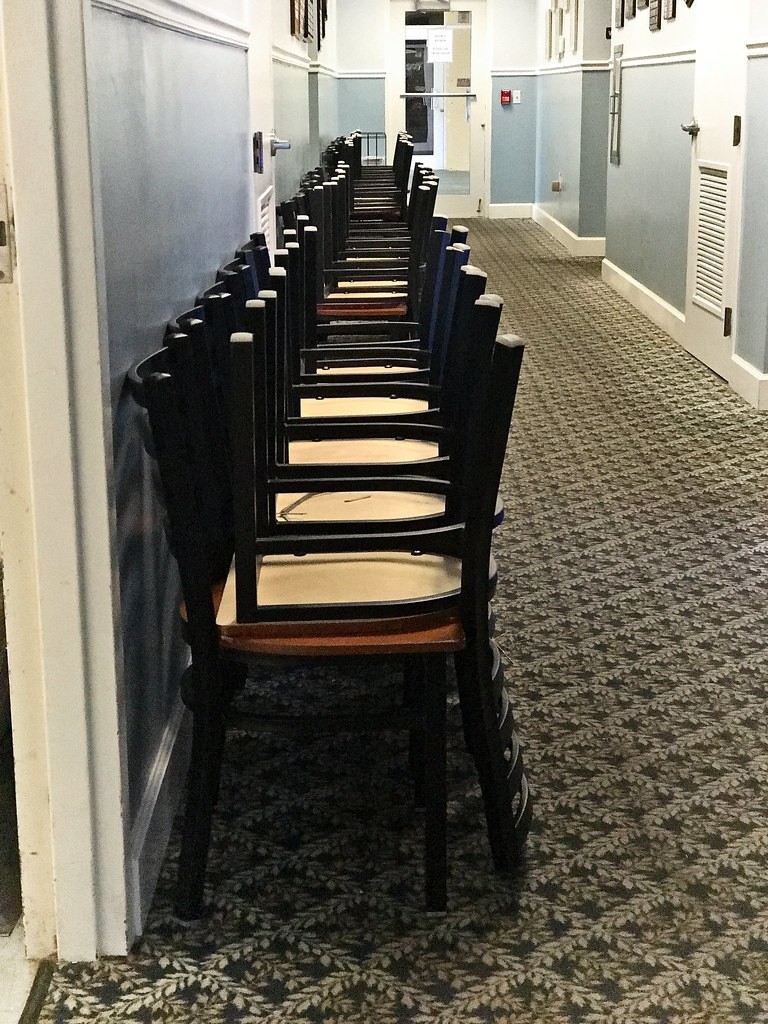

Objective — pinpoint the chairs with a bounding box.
[125,129,533,910]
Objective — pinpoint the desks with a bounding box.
[177,581,466,904]
